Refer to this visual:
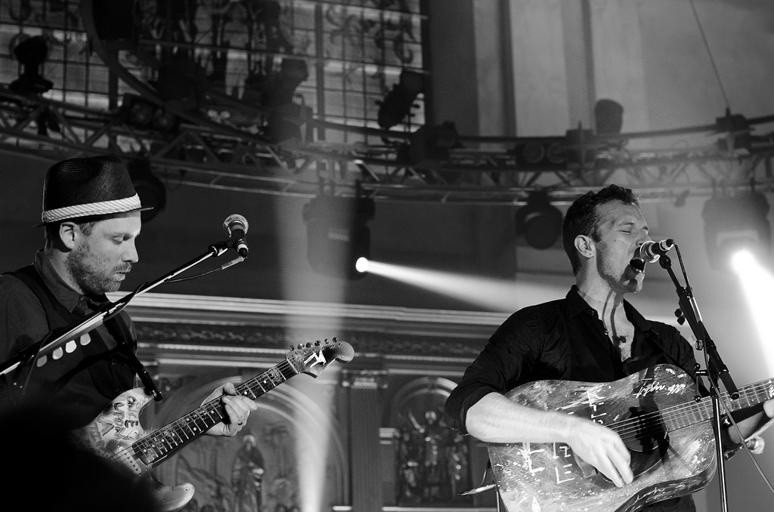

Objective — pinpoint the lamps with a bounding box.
[701,192,770,275]
[303,193,375,280]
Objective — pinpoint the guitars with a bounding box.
[51,337,354,511]
[488,364,774,512]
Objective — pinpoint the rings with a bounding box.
[237,422,243,425]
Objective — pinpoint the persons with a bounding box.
[1,150,259,511]
[446,184,774,512]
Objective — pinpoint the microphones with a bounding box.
[223,213,253,257]
[638,239,674,263]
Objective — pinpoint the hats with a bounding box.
[41,156,142,224]
